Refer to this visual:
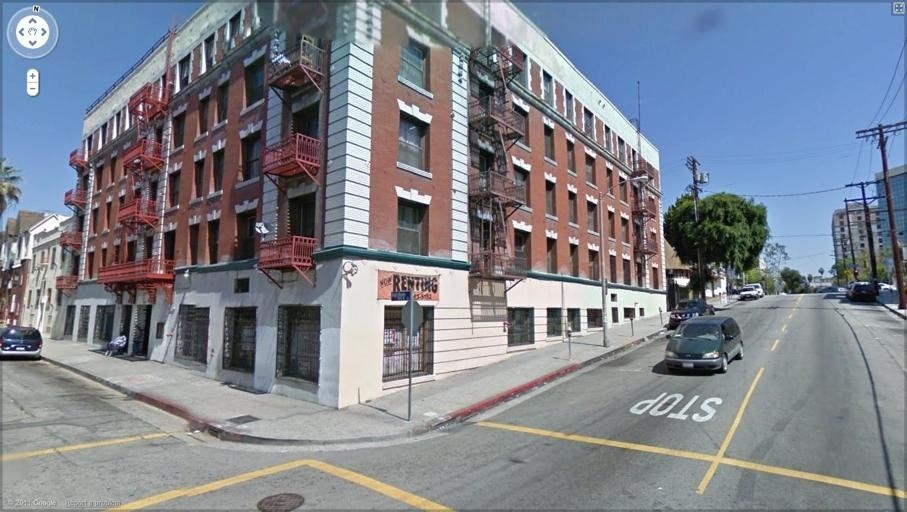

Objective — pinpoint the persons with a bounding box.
[104,330,126,357]
[130,323,143,356]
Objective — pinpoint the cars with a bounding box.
[829,280,896,301]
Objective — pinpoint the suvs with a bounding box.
[738,282,764,300]
[668,297,714,330]
[0,324,44,361]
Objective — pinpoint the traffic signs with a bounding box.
[391,292,409,300]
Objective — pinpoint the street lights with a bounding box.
[597,173,653,346]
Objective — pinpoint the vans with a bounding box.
[663,315,745,372]
